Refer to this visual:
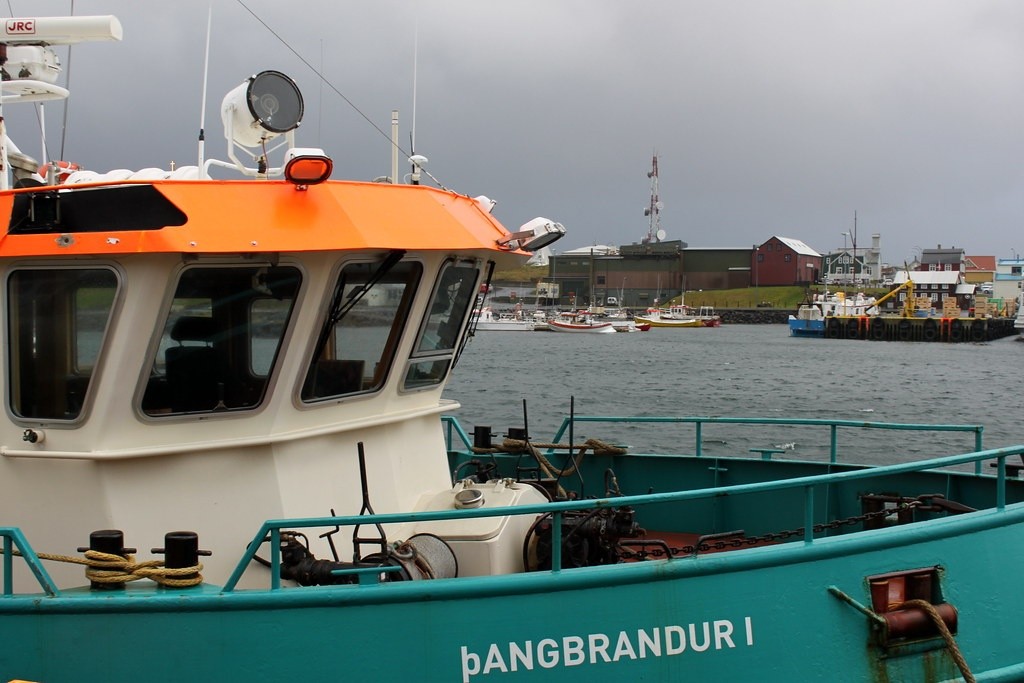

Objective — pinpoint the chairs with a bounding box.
[163,316,223,410]
[315,360,365,397]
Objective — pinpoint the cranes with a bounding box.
[863,279,916,320]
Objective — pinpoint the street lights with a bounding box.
[552,249,557,307]
[841,232,847,318]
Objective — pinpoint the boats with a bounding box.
[424,274,721,335]
[0,0,1024,683]
[788,288,826,338]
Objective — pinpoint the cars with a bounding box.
[480,283,494,295]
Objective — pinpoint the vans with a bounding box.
[607,296,617,305]
